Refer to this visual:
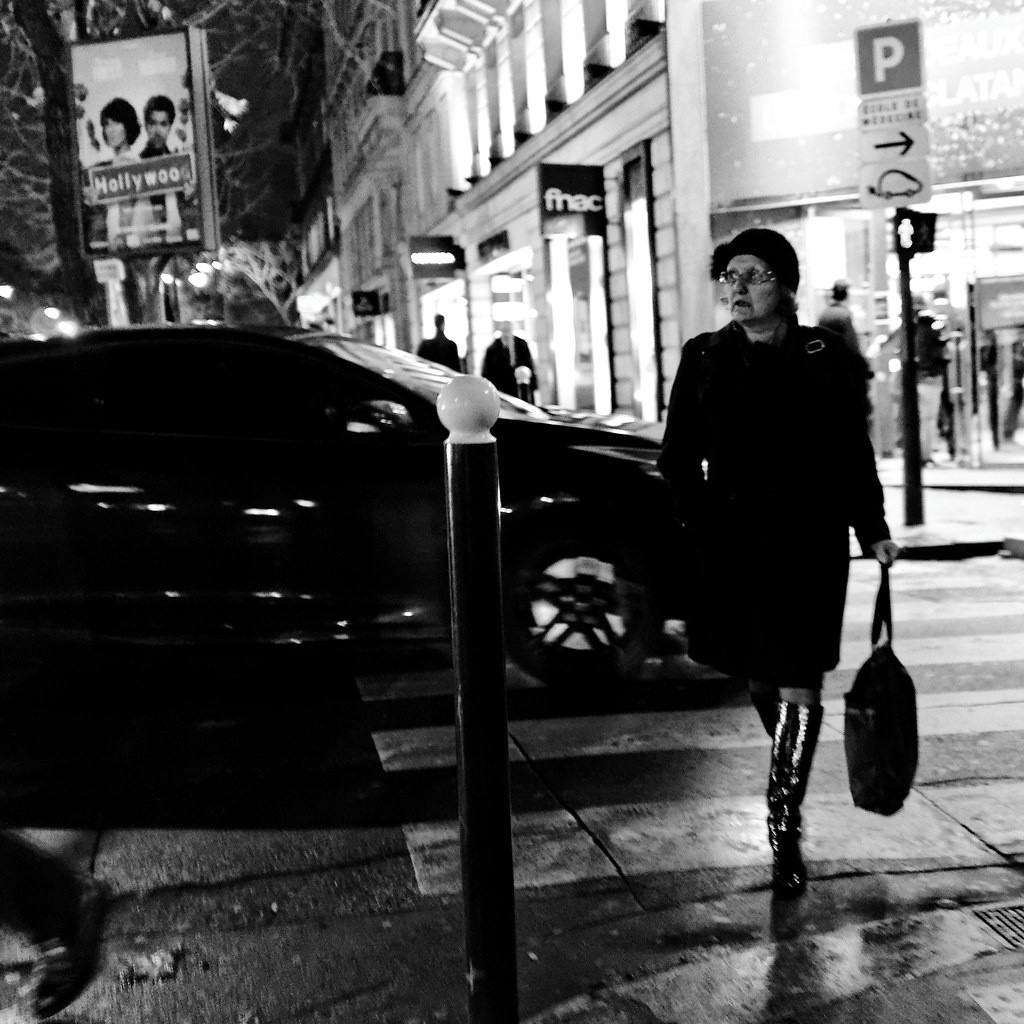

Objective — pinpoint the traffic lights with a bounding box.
[895,208,938,254]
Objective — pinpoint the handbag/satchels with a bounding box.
[843,551,918,817]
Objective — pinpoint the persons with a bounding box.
[0,827,114,1017]
[816,278,856,348]
[656,229,897,895]
[897,296,948,464]
[482,322,538,413]
[418,314,461,373]
[98,96,197,252]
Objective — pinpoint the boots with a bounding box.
[753,686,779,740]
[767,688,824,897]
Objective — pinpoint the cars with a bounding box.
[0,324,707,696]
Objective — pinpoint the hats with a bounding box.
[710,227,799,294]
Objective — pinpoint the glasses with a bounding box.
[719,268,779,286]
